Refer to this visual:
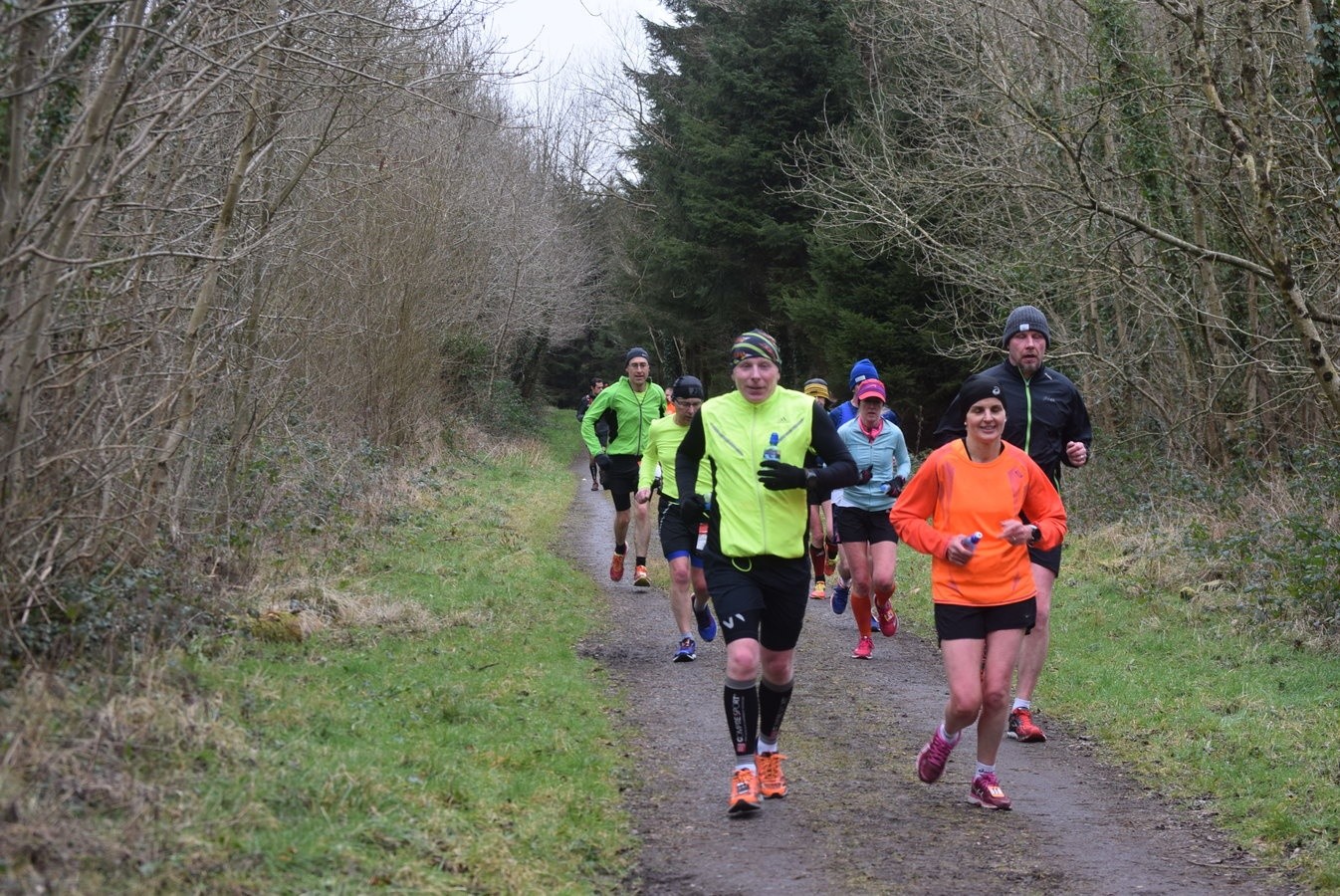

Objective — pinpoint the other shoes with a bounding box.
[592,482,599,491]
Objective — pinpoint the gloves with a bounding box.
[678,491,705,519]
[595,454,615,470]
[759,461,808,490]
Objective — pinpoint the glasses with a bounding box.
[673,397,702,408]
[627,363,650,370]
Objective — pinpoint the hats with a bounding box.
[625,347,651,369]
[1001,304,1051,351]
[850,359,882,392]
[672,375,704,399]
[803,378,832,407]
[728,327,782,376]
[957,373,1007,421]
[857,379,886,404]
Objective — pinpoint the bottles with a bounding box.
[881,485,891,493]
[762,432,782,478]
[960,531,982,551]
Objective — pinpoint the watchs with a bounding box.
[803,466,819,490]
[1028,523,1041,543]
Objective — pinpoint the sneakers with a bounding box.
[1007,706,1048,742]
[851,636,875,659]
[968,774,1013,810]
[825,544,838,576]
[609,541,628,581]
[831,577,853,616]
[874,594,898,636]
[871,611,881,632]
[915,720,963,784]
[632,565,651,587]
[810,581,827,600]
[728,737,788,814]
[671,592,718,661]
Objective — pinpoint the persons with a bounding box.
[662,386,683,418]
[820,357,899,634]
[580,346,668,588]
[635,376,718,664]
[889,374,1071,812]
[575,377,612,493]
[792,379,841,599]
[820,377,912,660]
[674,330,860,817]
[929,305,1094,744]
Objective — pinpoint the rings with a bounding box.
[1012,535,1017,542]
[1080,455,1084,460]
